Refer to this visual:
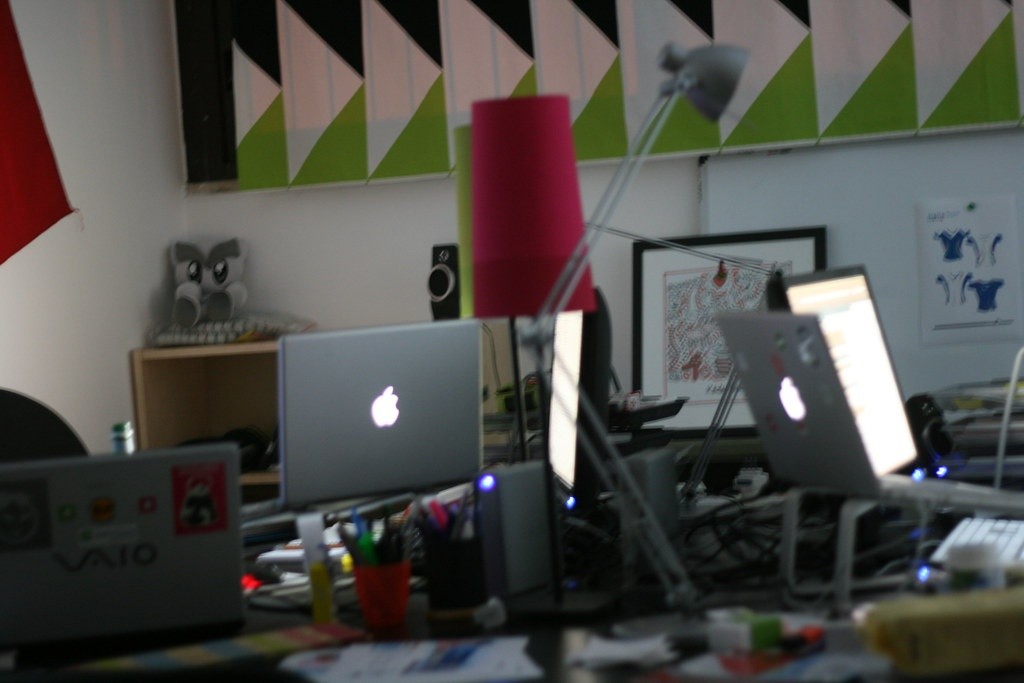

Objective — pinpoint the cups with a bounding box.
[351,560,410,629]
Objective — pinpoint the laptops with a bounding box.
[717,313,1023,517]
[241,317,485,522]
[0,440,247,652]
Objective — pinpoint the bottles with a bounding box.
[111,421,135,457]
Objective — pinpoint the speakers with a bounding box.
[427,243,460,320]
[904,392,955,464]
[474,459,552,597]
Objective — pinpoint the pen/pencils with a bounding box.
[338,488,470,568]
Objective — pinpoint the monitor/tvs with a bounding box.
[543,288,612,496]
[782,263,924,476]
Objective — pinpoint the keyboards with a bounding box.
[931,516,1024,577]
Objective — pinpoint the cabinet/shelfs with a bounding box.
[131,318,513,487]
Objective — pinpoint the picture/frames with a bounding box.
[632,224,827,440]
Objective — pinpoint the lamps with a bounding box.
[518,42,751,601]
[473,93,597,461]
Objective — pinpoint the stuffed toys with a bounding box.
[172,236,248,327]
